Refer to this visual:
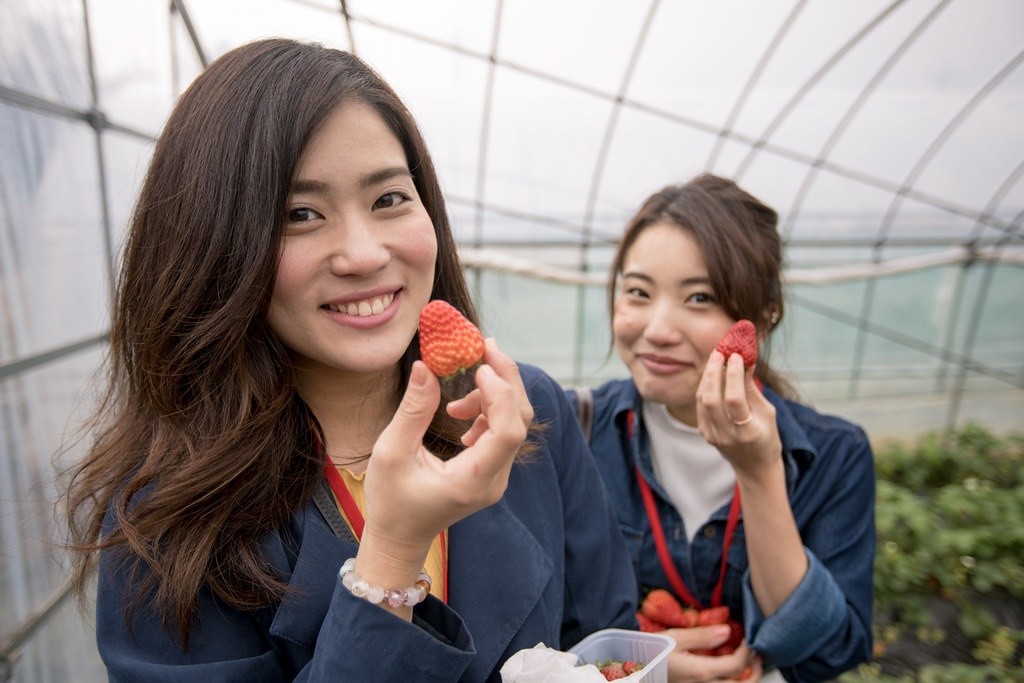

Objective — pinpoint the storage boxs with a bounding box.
[567,628,678,683]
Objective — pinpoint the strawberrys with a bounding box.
[633,589,762,681]
[715,318,758,371]
[420,299,485,380]
[592,658,646,682]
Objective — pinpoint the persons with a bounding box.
[67,38,638,682]
[562,171,876,683]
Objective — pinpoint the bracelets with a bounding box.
[339,557,433,609]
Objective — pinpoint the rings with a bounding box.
[732,413,752,427]
